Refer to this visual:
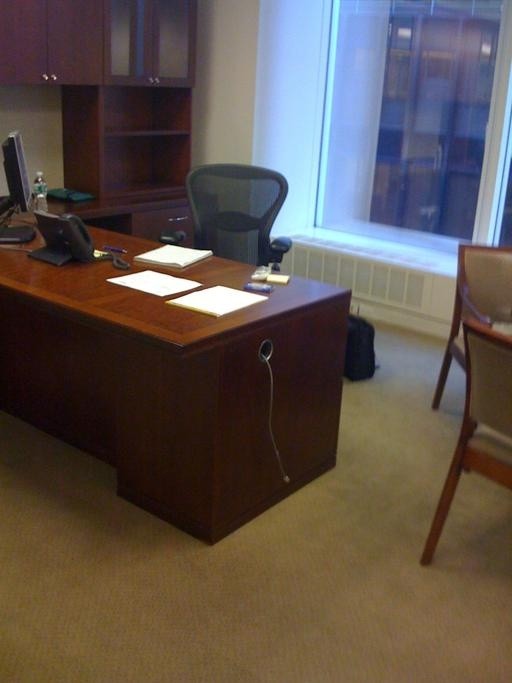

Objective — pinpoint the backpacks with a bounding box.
[343,311,377,380]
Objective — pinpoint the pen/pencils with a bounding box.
[104,246,128,254]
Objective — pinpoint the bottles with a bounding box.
[32,170,49,213]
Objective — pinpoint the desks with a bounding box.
[2,198,353,540]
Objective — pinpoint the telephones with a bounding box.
[27,209,93,266]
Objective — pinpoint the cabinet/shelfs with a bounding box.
[1,0,195,197]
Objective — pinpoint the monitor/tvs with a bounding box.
[0,129,35,243]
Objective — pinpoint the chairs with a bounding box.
[420,242,511,574]
[159,165,293,271]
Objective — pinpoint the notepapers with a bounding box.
[266,274,290,285]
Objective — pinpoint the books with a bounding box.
[166,284,269,318]
[105,270,203,298]
[134,244,213,269]
